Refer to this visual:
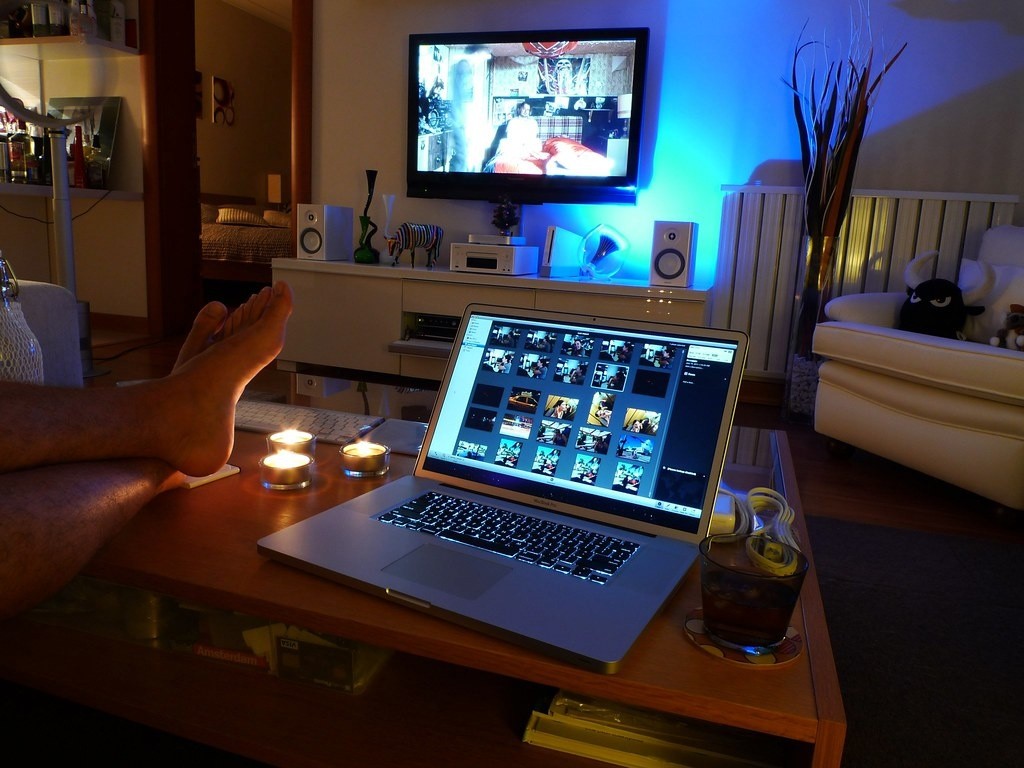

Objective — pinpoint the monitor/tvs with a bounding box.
[405,28,649,206]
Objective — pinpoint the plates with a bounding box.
[683,607,804,669]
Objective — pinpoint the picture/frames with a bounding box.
[38,97,121,187]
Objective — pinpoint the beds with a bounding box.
[200,195,294,285]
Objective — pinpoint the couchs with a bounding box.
[810,224,1024,511]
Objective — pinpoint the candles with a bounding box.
[258,450,316,490]
[265,428,317,455]
[339,440,392,475]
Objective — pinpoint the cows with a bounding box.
[384,222,444,270]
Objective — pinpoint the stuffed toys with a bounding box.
[895,251,995,339]
[990,304,1024,352]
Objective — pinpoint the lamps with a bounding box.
[268,174,287,208]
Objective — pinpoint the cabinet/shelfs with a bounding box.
[0,36,144,211]
[266,253,716,418]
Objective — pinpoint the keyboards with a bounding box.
[233,399,386,446]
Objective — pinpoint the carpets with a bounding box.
[805,509,1024,768]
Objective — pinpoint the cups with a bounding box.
[699,534,808,652]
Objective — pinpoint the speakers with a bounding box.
[650,221,699,287]
[297,205,354,261]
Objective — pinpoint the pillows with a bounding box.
[202,203,217,223]
[216,207,263,226]
[262,209,291,228]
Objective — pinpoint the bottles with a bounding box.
[69,126,110,189]
[0,106,43,183]
[30,0,126,46]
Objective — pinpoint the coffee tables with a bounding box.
[1,369,849,768]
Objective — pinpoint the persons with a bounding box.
[505,102,539,138]
[0,280,293,618]
[458,326,674,492]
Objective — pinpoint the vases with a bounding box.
[785,235,842,417]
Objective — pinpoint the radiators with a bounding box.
[714,185,1019,478]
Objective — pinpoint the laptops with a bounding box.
[256,302,750,676]
